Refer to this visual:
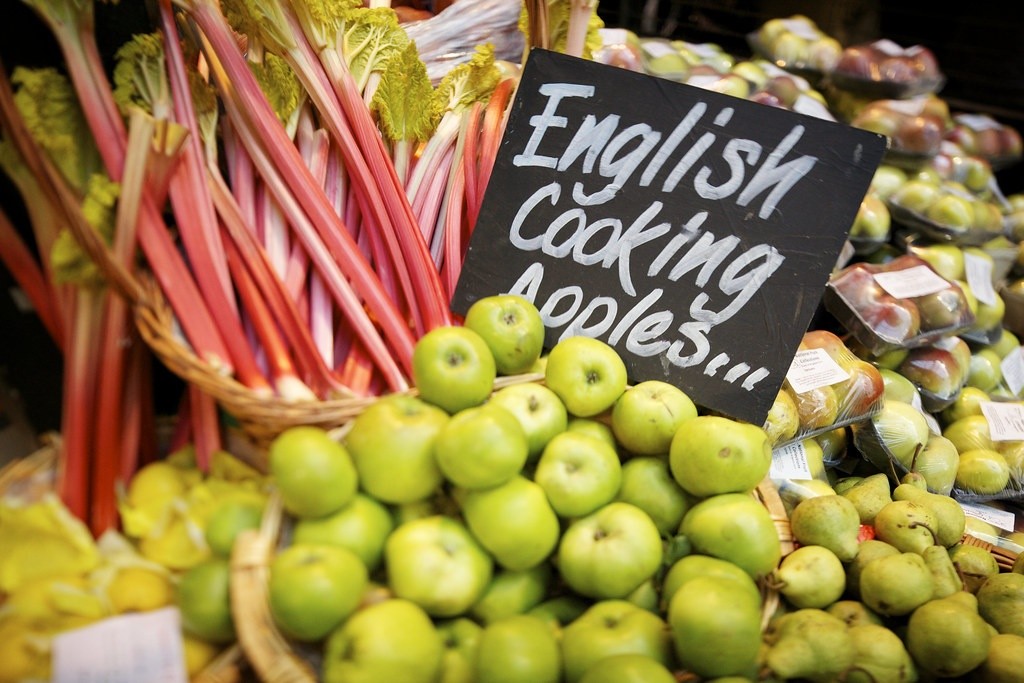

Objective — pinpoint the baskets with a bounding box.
[0,0,1024,683]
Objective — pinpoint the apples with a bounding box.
[176,293,780,683]
[591,15,1024,497]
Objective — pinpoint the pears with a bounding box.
[759,442,1024,683]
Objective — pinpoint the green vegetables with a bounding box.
[0,0,598,538]
